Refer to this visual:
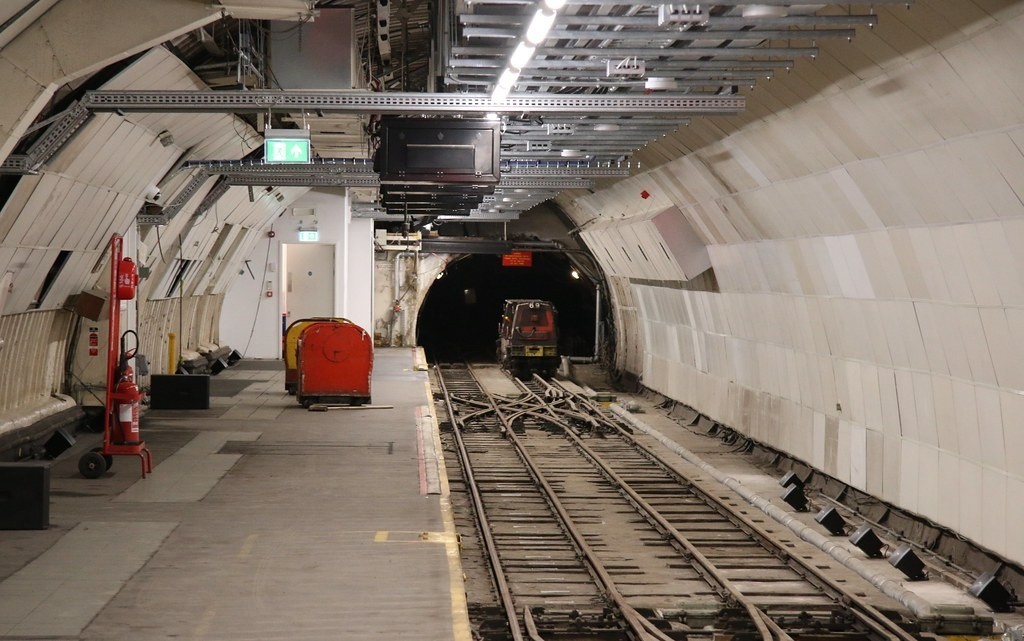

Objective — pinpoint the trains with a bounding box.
[495,298,559,381]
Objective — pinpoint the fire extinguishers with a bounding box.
[112,375,141,445]
[114,329,140,393]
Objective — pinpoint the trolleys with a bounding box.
[77,234,153,480]
